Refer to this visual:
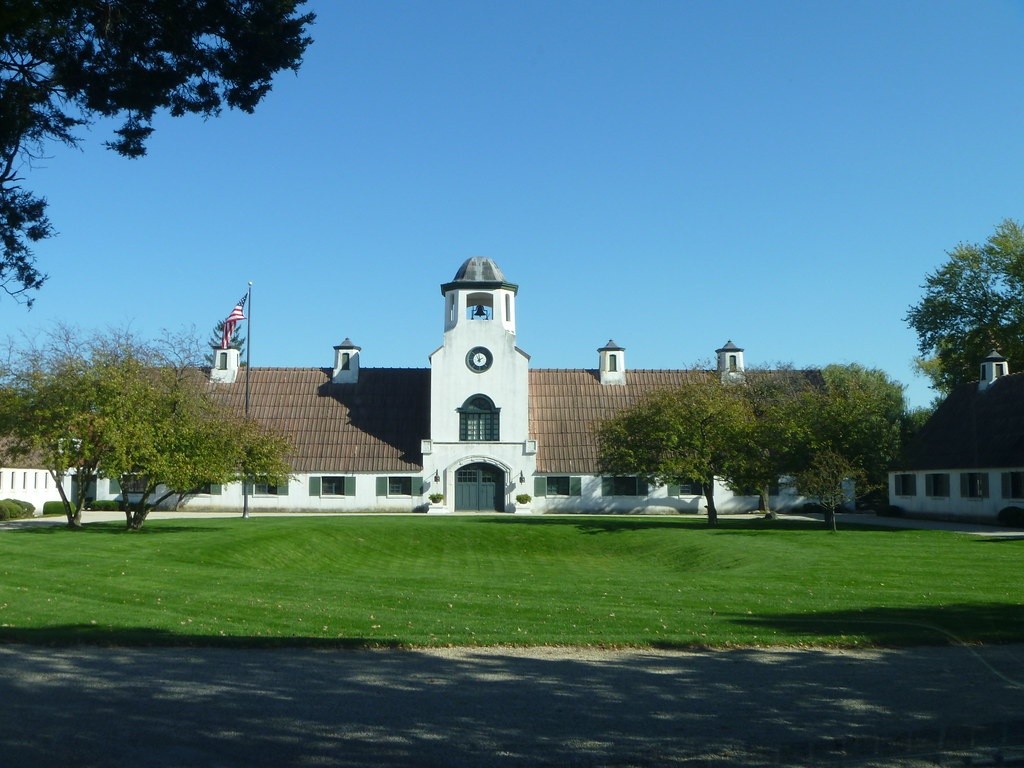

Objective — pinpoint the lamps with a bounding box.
[511,470,525,485]
[424,469,440,484]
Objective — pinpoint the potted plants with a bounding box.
[516,494,531,504]
[429,493,444,503]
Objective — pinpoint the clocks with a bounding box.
[473,353,486,367]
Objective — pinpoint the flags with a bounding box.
[222,293,248,350]
